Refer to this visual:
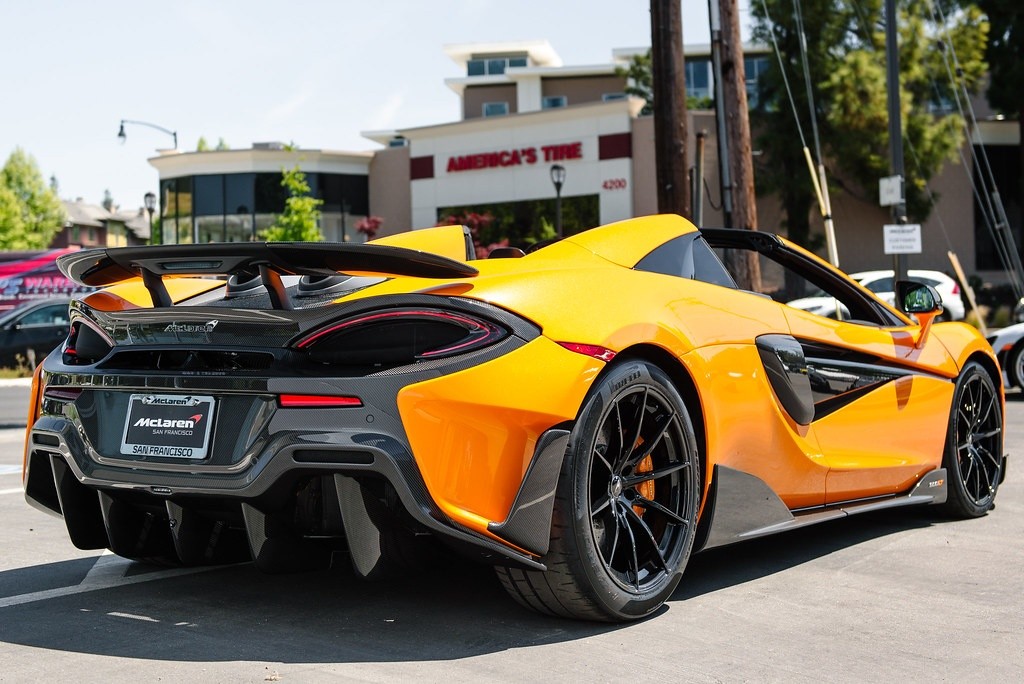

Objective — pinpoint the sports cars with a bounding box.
[18,212,1011,622]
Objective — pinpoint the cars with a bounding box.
[782,269,965,328]
[0,248,110,367]
[986,297,1024,398]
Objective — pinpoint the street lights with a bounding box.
[550,164,568,241]
[143,193,158,248]
[116,117,181,152]
